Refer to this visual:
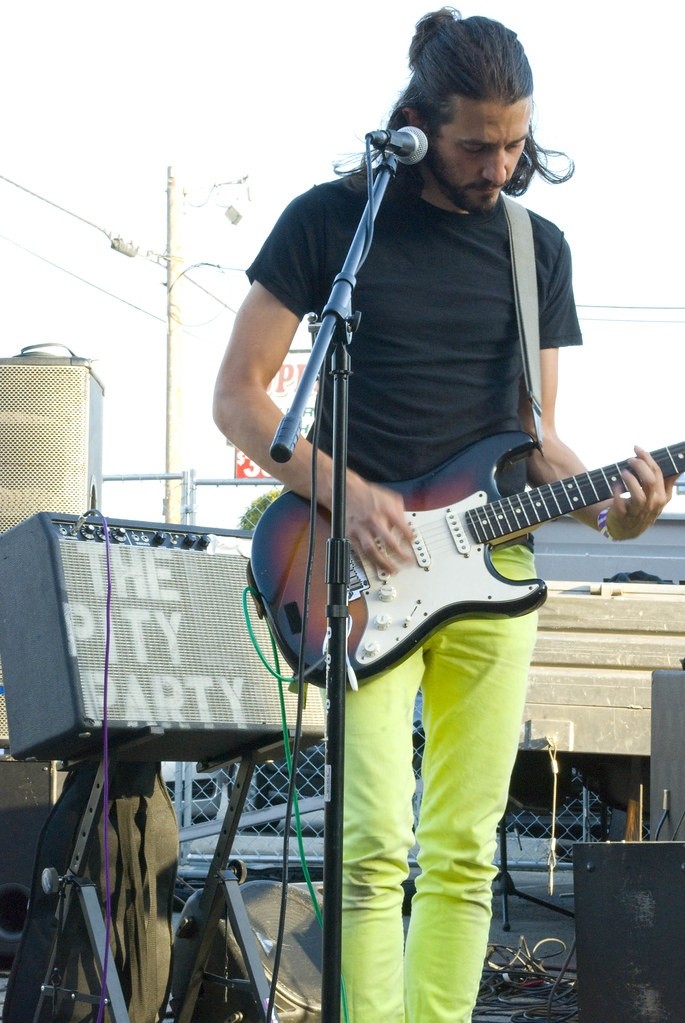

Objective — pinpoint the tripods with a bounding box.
[489,812,577,931]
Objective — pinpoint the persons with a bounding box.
[214,10,680,1022]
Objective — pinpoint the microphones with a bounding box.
[364,126,429,166]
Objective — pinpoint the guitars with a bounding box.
[245,441,685,693]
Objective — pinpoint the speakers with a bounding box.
[648,667,684,844]
[0,758,61,971]
[570,839,685,1023]
[0,511,325,768]
[167,879,330,1023]
[0,351,107,748]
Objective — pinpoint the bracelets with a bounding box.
[597,507,616,542]
